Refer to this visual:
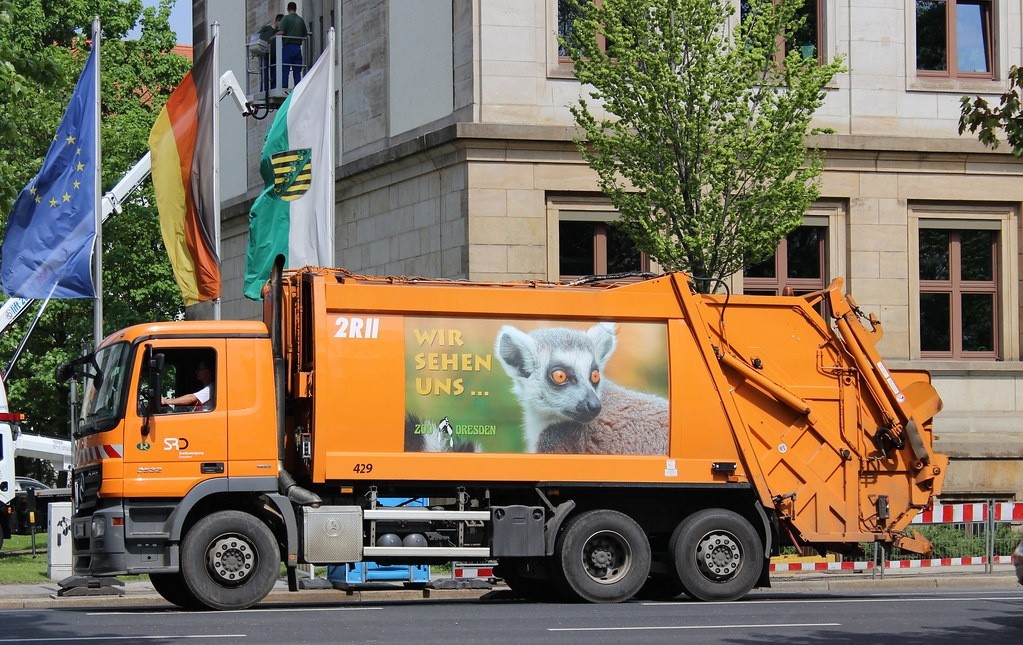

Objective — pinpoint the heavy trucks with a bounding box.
[43,252,950,612]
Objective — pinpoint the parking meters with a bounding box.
[25,485,37,556]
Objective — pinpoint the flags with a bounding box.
[243,47,333,302]
[148,37,222,306]
[0,32,99,298]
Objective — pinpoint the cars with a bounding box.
[9,472,54,535]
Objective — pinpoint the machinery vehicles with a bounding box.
[0,32,310,554]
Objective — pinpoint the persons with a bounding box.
[258,14,284,92]
[161,360,214,411]
[270,2,308,89]
